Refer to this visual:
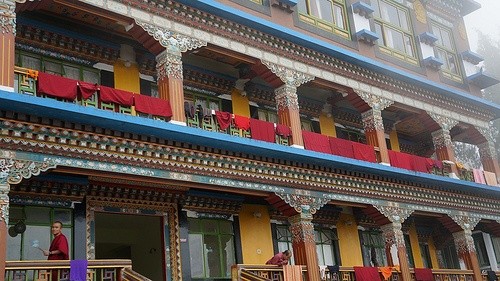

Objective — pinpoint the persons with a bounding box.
[266,250,293,281]
[43,221,70,281]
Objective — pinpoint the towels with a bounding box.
[70,259,88,281]
[484,170,498,186]
[472,169,486,185]
[283,264,303,281]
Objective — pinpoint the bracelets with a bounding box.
[50,251,52,255]
